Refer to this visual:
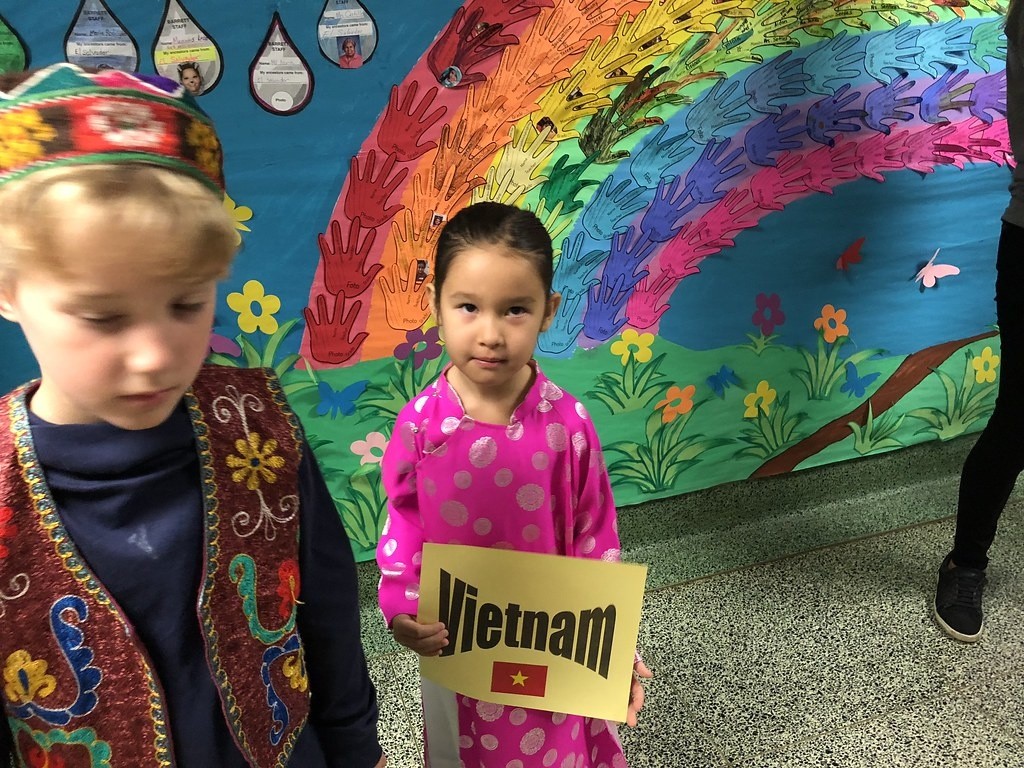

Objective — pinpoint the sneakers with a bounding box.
[934,548,988,643]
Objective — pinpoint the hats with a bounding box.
[0,61,227,201]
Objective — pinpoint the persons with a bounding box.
[372,201,655,768]
[1,65,385,768]
[936,0,1024,642]
